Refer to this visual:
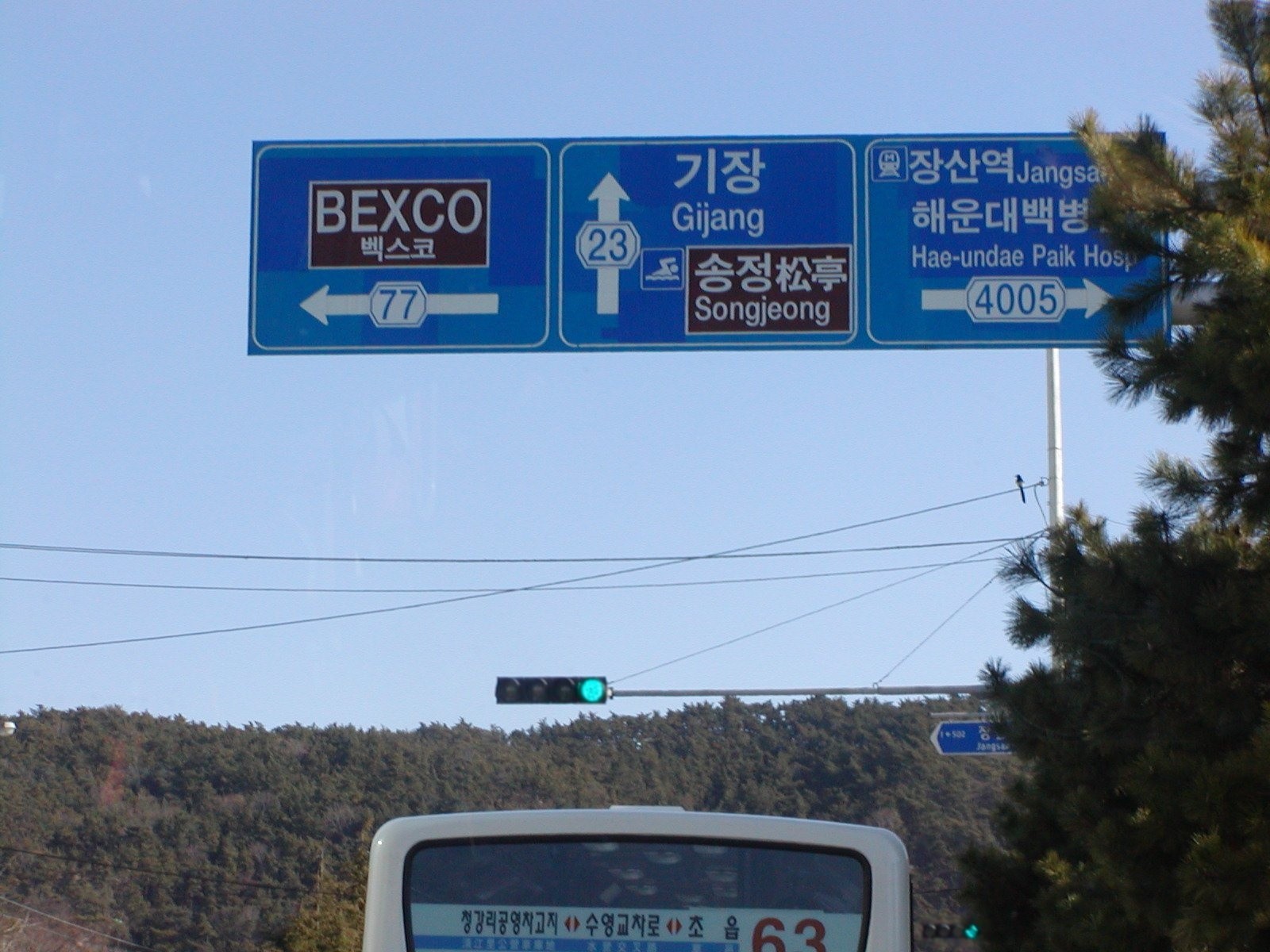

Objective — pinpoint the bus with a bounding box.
[363,805,913,952]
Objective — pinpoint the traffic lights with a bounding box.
[921,922,983,939]
[494,676,608,705]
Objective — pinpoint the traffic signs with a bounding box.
[932,721,1031,756]
[553,136,865,349]
[249,138,554,356]
[857,133,1173,350]
